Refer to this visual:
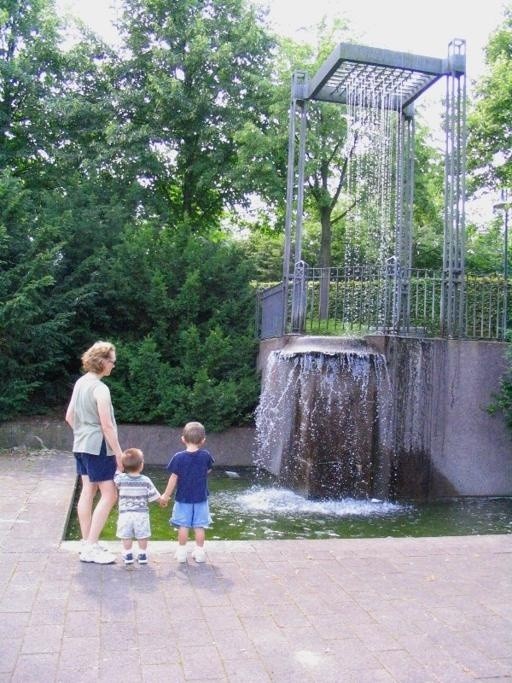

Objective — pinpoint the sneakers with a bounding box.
[192,550,205,563]
[121,553,134,564]
[78,541,117,564]
[137,554,148,564]
[176,550,187,563]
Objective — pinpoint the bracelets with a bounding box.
[163,493,171,500]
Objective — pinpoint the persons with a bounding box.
[112,448,165,564]
[159,421,214,563]
[64,341,124,564]
